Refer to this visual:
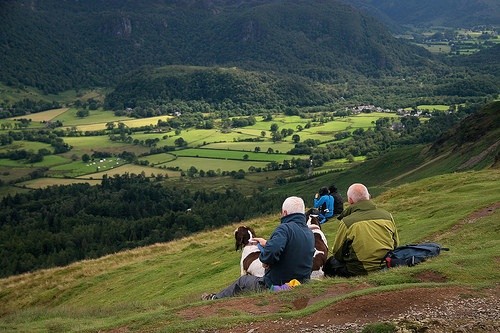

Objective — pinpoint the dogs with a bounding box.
[306,214,328,279]
[234,226,265,277]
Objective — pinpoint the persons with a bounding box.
[305,186,334,218]
[322,183,400,279]
[200,196,315,301]
[328,185,343,215]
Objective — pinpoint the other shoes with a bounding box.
[201,290,216,301]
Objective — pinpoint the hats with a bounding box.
[318,187,330,194]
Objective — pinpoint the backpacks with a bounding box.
[380,243,451,270]
